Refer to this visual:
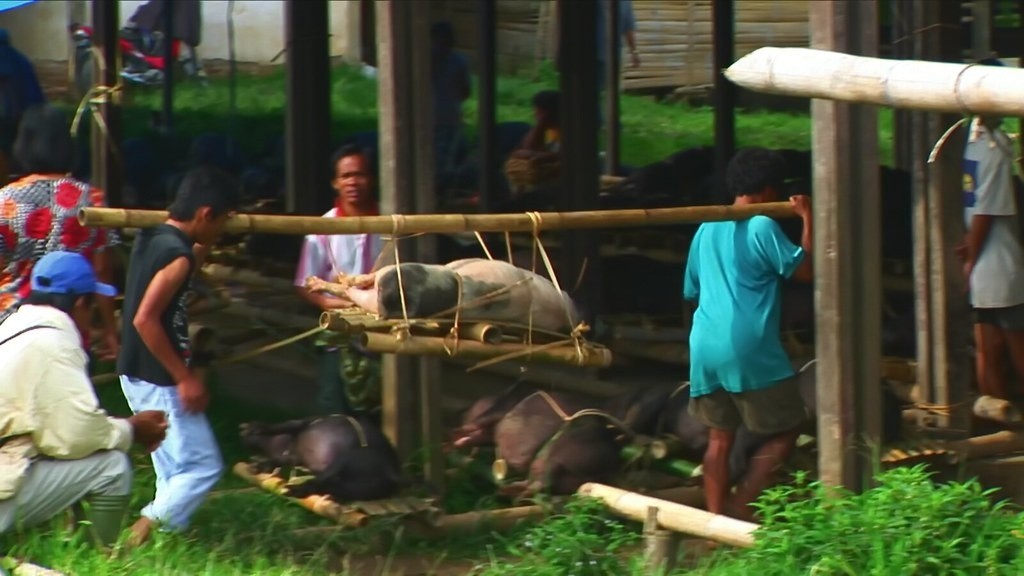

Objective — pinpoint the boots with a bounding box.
[87,494,132,542]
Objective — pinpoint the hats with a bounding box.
[30,250,116,297]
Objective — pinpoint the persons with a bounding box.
[117,165,234,548]
[512,91,563,164]
[682,145,814,547]
[954,57,1024,399]
[619,2,643,68]
[430,20,471,188]
[0,100,121,363]
[0,28,47,147]
[239,142,397,312]
[0,250,170,549]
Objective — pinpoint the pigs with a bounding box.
[307,259,583,333]
[238,348,918,500]
